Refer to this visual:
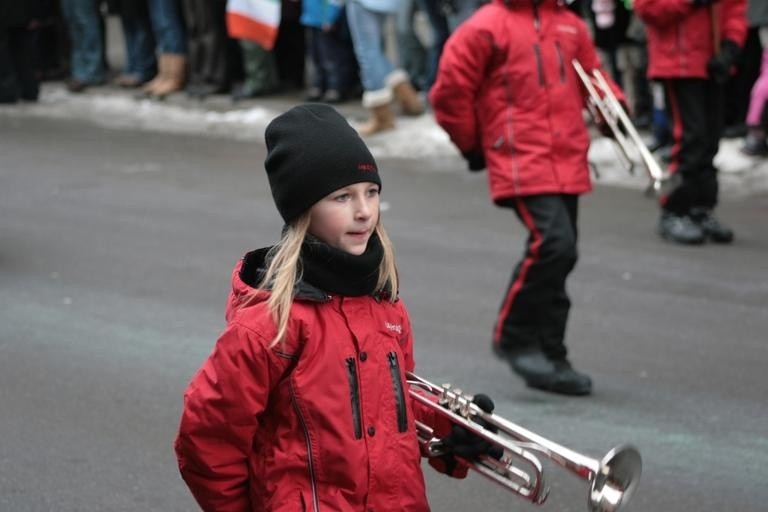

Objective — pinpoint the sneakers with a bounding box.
[303,87,349,103]
[113,71,144,88]
[657,208,734,246]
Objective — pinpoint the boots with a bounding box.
[187,35,278,98]
[359,87,399,139]
[138,52,186,96]
[384,67,424,116]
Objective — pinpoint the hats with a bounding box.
[264,103,381,226]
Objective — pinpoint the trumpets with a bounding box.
[571,55,684,202]
[404,370,642,512]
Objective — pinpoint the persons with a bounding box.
[630,0,750,250]
[173,103,503,509]
[429,0,632,396]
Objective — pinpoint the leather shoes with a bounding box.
[495,332,595,397]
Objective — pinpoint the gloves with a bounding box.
[460,146,487,173]
[705,36,742,84]
[451,393,508,461]
[598,107,629,139]
[693,0,718,8]
